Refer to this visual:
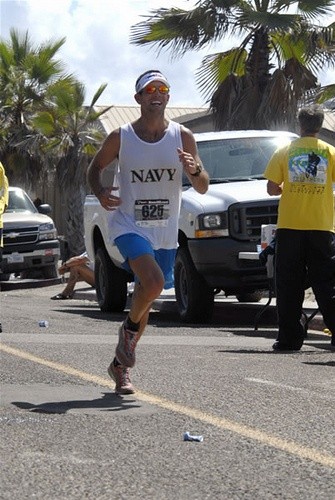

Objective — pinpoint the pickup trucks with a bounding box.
[84,130,304,325]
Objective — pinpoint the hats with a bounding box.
[136,72,171,93]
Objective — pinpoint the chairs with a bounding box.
[250,153,273,176]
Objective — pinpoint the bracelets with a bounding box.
[63,262,69,268]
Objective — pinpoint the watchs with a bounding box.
[190,163,202,177]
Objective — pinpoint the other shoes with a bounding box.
[272,342,303,350]
[50,293,71,299]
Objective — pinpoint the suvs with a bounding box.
[0,186,61,281]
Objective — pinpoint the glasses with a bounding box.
[145,85,169,94]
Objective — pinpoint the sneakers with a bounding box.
[115,313,139,368]
[107,357,135,394]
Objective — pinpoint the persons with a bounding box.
[50,251,95,300]
[0,162,9,333]
[87,70,209,395]
[264,106,335,351]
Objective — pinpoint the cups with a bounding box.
[257,245,261,252]
